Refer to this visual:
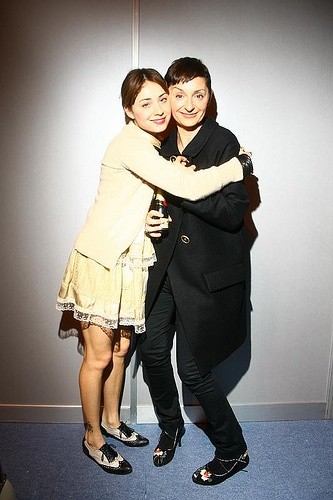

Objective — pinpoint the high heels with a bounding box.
[153,426,186,466]
[192,450,249,485]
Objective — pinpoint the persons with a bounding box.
[144,58,251,485]
[56,68,255,474]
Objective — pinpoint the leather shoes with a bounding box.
[100,419,149,447]
[82,437,132,475]
